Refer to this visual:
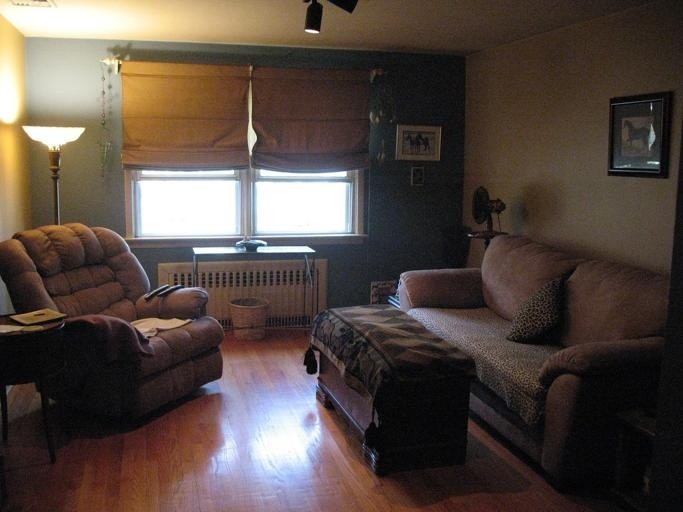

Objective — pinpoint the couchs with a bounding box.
[400,234,670,491]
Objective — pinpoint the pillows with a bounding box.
[481,234,586,321]
[557,258,671,349]
[505,277,566,340]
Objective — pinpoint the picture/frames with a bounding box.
[395,123,442,161]
[607,91,669,178]
[411,167,425,185]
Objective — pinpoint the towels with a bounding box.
[65,313,155,355]
[130,316,196,338]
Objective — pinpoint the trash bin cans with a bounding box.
[228,296,270,341]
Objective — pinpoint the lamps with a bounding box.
[22,124,85,226]
[303,0,358,34]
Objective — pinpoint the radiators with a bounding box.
[158,258,330,331]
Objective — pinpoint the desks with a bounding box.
[192,245,317,328]
[388,295,400,308]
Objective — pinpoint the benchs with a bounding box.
[314,303,476,475]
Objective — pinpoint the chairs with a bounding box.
[0,222,224,430]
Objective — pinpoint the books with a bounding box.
[9,308,67,325]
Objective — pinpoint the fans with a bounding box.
[468,186,507,245]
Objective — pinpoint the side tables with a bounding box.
[0,314,72,463]
